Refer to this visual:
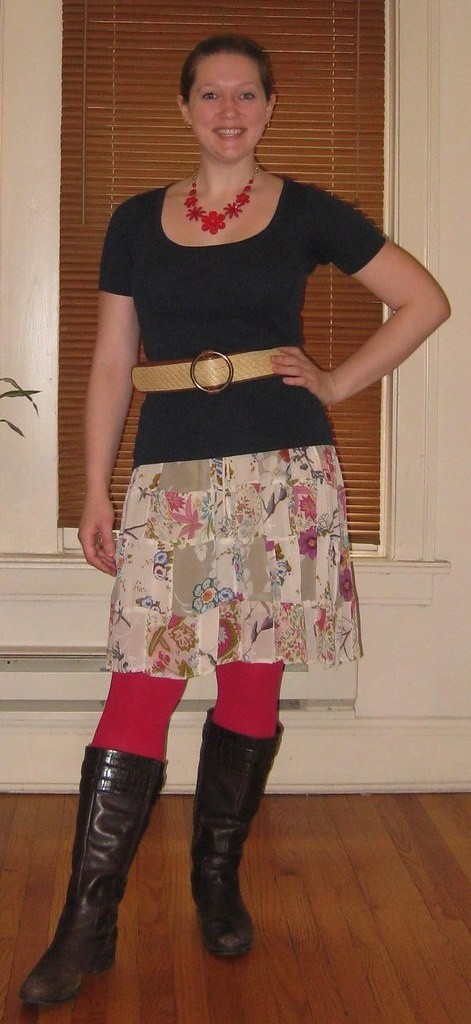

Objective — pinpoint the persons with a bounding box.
[16,36,451,1003]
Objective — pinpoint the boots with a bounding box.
[188,705,284,957]
[16,743,167,1007]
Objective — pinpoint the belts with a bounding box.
[130,344,288,396]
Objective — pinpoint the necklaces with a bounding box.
[184,165,259,234]
